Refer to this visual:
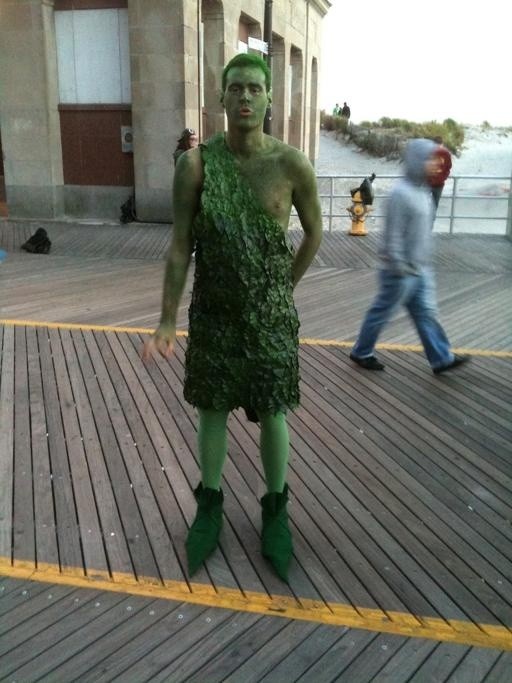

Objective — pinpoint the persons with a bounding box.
[347,138,472,375]
[425,136,452,227]
[144,53,323,584]
[172,128,198,257]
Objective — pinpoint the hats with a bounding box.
[177,128,195,142]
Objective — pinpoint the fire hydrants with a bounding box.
[345,190,376,236]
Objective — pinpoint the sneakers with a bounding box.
[431,351,473,375]
[349,350,386,370]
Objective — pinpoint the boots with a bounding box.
[183,480,224,578]
[259,481,295,584]
